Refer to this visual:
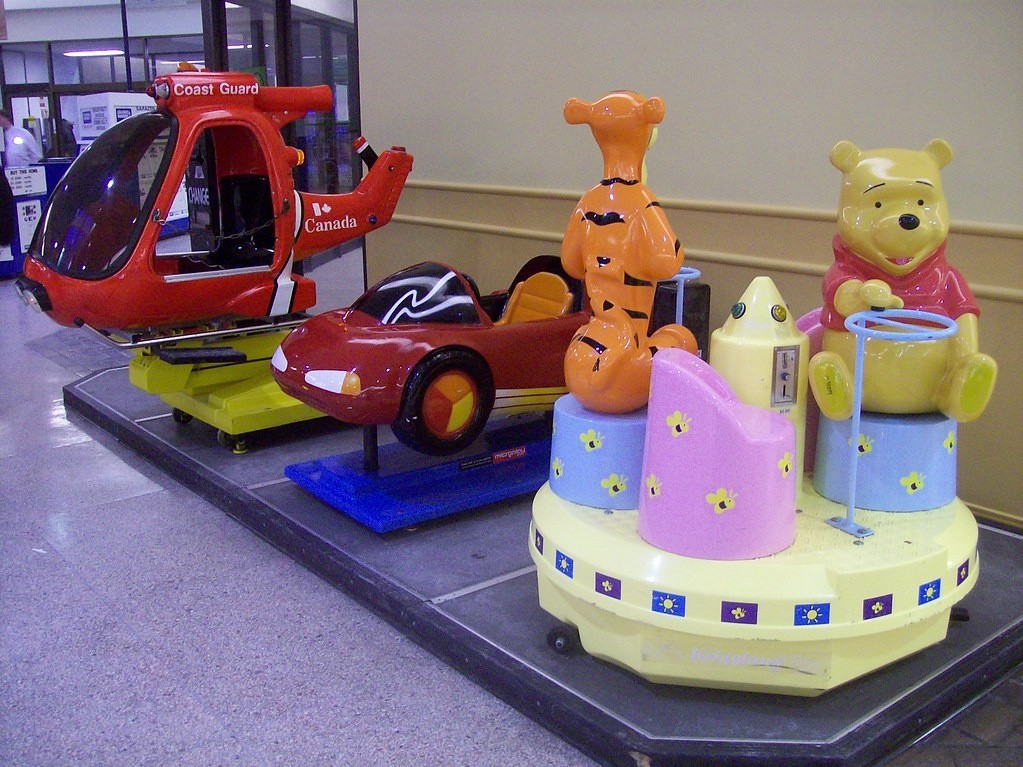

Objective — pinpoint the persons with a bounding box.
[62,119,76,156]
[0,109,43,167]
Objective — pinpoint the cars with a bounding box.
[272,254,712,456]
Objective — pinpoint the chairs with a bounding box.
[176,169,274,279]
[493,272,575,332]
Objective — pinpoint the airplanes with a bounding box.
[13,59,416,335]
